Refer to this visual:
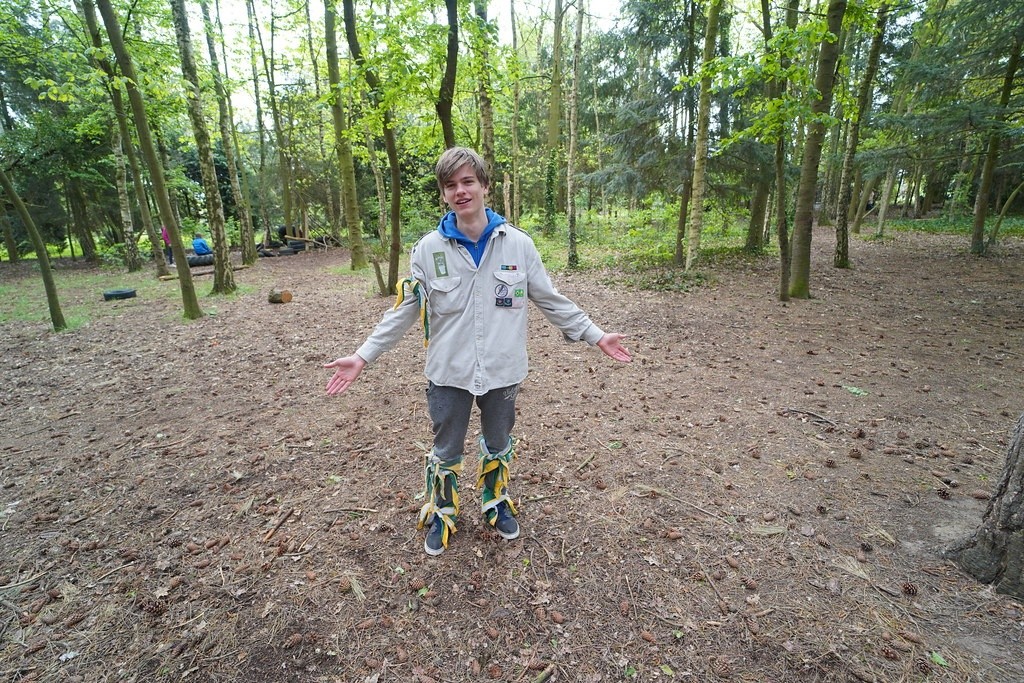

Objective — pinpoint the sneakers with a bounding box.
[486,505,520,539]
[424,516,450,555]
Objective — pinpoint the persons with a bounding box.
[192,233,215,257]
[323,147,631,556]
[162,228,173,264]
